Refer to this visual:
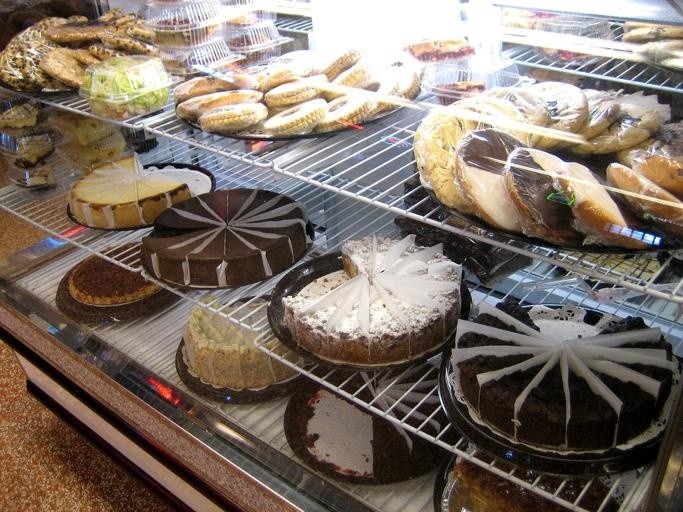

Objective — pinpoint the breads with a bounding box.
[0,8,683,263]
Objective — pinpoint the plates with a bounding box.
[174,296,302,406]
[269,247,468,365]
[65,163,214,232]
[53,264,187,320]
[448,207,683,257]
[173,82,420,143]
[433,455,636,512]
[141,220,317,290]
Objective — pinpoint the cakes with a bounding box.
[182,290,307,392]
[441,443,639,512]
[67,241,164,308]
[141,188,316,292]
[281,233,465,368]
[70,155,191,229]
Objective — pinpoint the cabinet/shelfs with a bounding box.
[0,0,681,512]
